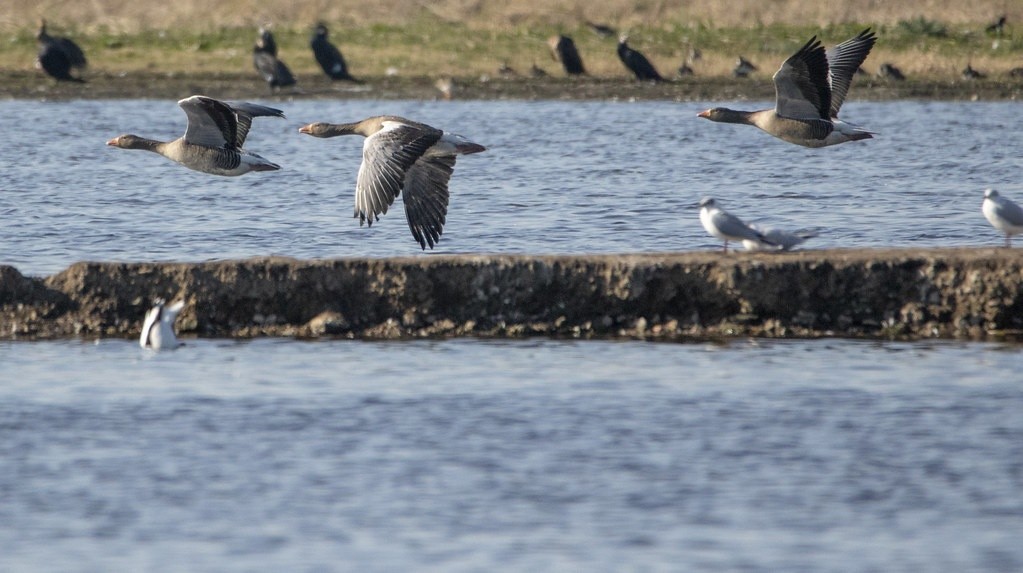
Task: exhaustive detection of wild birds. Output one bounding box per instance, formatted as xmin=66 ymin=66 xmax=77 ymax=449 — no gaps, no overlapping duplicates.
xmin=981 ymin=188 xmax=1023 ymax=248
xmin=311 ymin=23 xmax=367 ymax=88
xmin=697 ymin=28 xmax=879 ymax=148
xmin=34 ymin=14 xmax=90 ymax=84
xmin=299 ymin=115 xmax=486 ymax=250
xmin=139 ymin=296 xmax=185 ymax=349
xmin=107 ymin=95 xmax=287 ymax=176
xmin=698 ymin=195 xmax=821 ymax=252
xmin=252 ymin=23 xmax=298 ymax=96
xmin=617 ymin=33 xmax=662 ymax=80
